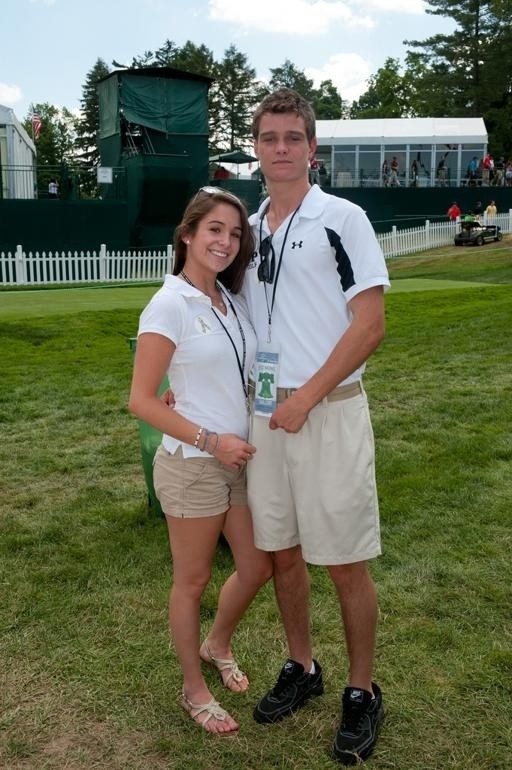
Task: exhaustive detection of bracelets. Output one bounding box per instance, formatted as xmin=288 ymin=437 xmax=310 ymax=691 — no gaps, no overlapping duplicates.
xmin=195 ymin=426 xmax=220 ymax=455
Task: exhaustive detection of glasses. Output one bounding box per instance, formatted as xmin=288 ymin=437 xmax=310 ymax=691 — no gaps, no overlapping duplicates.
xmin=257 ymin=234 xmax=276 ymax=284
xmin=191 ymin=185 xmax=224 ymax=203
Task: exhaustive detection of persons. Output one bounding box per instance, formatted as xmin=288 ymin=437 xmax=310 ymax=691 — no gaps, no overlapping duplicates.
xmin=381 ymin=154 xmax=402 ymax=188
xmin=460 ymin=154 xmax=511 ymax=189
xmin=240 ymin=86 xmax=385 ymax=765
xmin=307 ymin=155 xmax=328 ymax=187
xmin=411 ymin=160 xmax=418 ymax=187
xmin=446 ymin=199 xmax=498 ymax=231
xmin=438 ymin=155 xmax=449 ymax=187
xmin=125 ymin=186 xmax=276 ymax=737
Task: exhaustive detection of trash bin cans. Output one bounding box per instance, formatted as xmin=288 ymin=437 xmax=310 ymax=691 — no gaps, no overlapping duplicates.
xmin=125 ymin=338 xmax=171 ymax=517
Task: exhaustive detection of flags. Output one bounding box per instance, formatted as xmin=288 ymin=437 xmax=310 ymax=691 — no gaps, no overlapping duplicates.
xmin=32 ymin=105 xmax=43 ymax=141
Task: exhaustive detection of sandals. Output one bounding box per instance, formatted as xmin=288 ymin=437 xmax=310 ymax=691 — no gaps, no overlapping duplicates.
xmin=197 ymin=634 xmax=251 ymax=693
xmin=180 ymin=682 xmax=239 ymax=735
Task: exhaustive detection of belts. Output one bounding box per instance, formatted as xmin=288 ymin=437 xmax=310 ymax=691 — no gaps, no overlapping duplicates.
xmin=247 ymin=375 xmax=361 ymax=405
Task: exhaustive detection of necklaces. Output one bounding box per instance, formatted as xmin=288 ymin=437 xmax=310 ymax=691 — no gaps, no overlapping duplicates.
xmin=177 ymin=267 xmax=229 ymax=314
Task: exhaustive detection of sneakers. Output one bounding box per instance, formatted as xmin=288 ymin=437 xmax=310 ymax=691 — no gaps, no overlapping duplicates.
xmin=333 ymin=682 xmax=386 ymax=763
xmin=251 ymin=657 xmax=324 ymax=725
